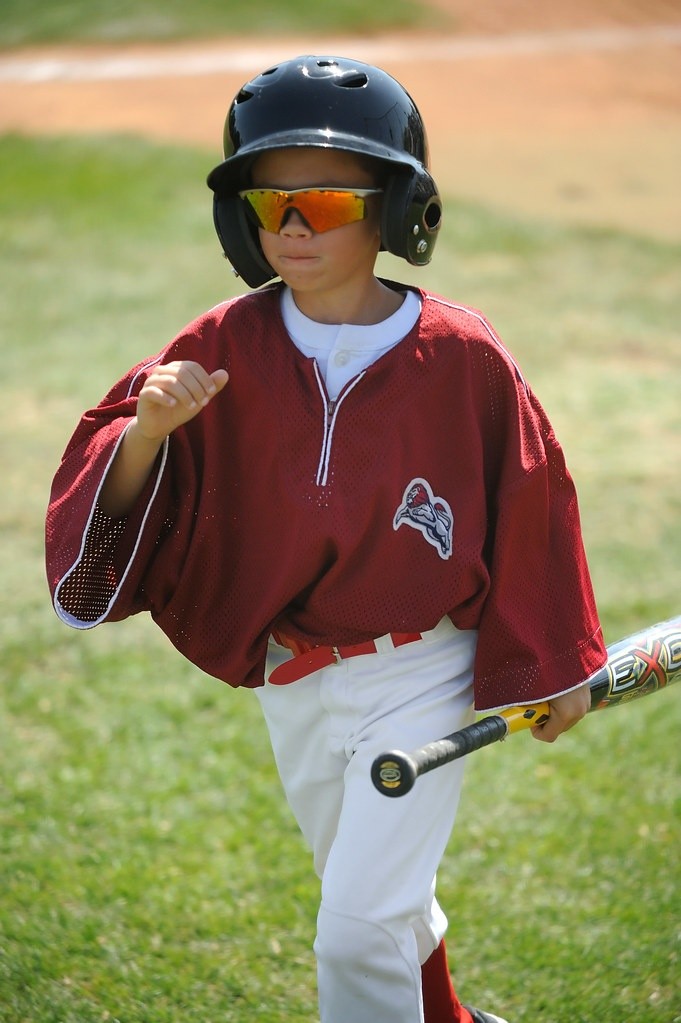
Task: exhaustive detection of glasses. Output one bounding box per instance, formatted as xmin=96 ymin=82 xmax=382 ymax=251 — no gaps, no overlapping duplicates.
xmin=237 ymin=185 xmax=387 ymax=235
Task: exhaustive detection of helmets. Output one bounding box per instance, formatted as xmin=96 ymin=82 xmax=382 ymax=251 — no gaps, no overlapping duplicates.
xmin=205 ymin=55 xmax=442 ymax=289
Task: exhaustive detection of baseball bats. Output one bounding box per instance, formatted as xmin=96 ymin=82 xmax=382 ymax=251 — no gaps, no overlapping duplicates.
xmin=370 ymin=614 xmax=681 ymax=798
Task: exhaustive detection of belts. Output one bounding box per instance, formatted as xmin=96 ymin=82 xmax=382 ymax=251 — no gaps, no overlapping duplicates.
xmin=268 ymin=626 xmax=423 ymax=686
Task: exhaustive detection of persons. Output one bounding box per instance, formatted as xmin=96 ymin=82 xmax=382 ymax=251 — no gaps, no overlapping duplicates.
xmin=46 ymin=56 xmax=607 ymax=1023
